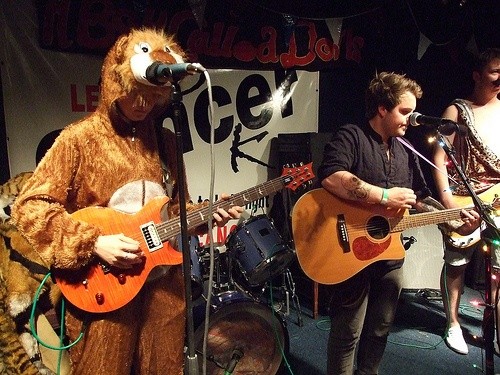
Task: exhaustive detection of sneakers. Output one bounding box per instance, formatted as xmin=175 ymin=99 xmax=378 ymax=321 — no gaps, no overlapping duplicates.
xmin=445 ymin=324 xmax=468 ymax=355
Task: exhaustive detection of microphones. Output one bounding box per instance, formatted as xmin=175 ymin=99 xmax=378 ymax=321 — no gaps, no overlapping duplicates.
xmin=409 ymin=112 xmax=458 ymax=128
xmin=146 ymin=61 xmax=205 ymax=86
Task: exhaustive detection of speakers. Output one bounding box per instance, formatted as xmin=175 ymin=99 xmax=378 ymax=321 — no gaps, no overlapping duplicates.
xmin=278 ymin=132 xmax=334 ymax=237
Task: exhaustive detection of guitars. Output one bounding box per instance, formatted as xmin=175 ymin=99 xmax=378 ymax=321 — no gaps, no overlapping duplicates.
xmin=55 ymin=161 xmax=315 ymax=313
xmin=292 ymin=187 xmax=500 ymax=294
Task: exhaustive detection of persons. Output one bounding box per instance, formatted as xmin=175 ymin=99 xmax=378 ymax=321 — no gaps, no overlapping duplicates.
xmin=315 ymin=69 xmax=482 ymax=375
xmin=9 ymin=26 xmax=244 ymax=374
xmin=432 ymin=48 xmax=500 ymax=356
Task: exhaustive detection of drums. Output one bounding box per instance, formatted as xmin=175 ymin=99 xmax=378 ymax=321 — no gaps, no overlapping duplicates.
xmin=225 ymin=214 xmax=292 ymax=287
xmin=183 ymin=289 xmax=290 ymax=375
xmin=178 ymin=234 xmax=204 ymax=301
